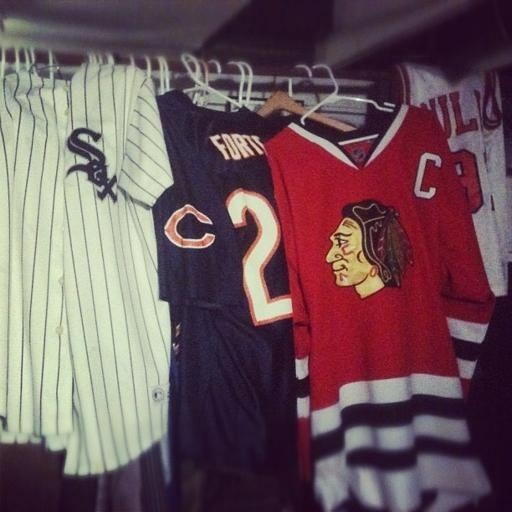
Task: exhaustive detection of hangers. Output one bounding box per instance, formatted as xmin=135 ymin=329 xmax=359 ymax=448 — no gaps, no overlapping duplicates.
xmin=300 ymin=63 xmax=394 ymax=147
xmin=1 ymin=40 xmax=357 ymax=149
